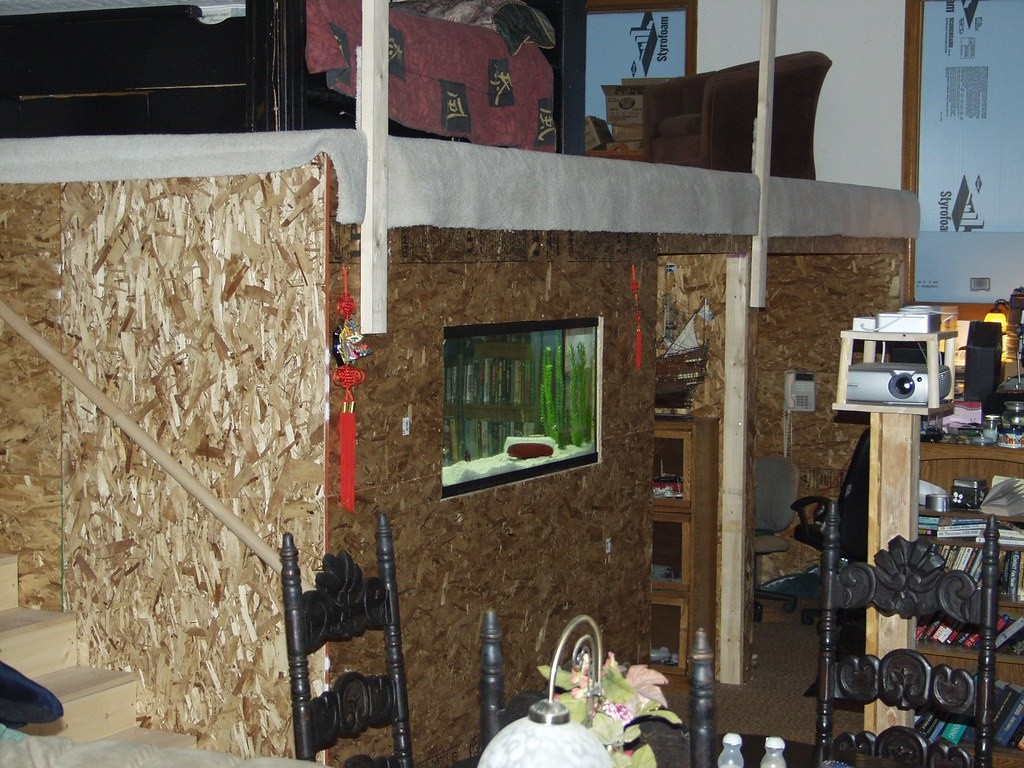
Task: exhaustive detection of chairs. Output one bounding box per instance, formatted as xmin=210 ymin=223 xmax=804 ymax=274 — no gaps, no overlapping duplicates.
xmin=280 ymin=512 xmax=414 ymax=768
xmin=478 ymin=608 xmax=717 ymax=768
xmin=815 ymin=501 xmax=1000 ymax=768
xmin=752 ymin=456 xmax=799 ymax=622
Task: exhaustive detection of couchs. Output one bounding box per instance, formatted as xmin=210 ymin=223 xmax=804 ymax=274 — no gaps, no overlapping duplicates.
xmin=642 ymin=51 xmax=833 ymax=180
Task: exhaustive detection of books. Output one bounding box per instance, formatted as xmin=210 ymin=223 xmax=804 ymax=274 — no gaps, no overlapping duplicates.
xmin=918 ymin=516 xmax=1024 ymax=545
xmin=913 ymin=671 xmax=1024 ymax=750
xmin=980 ymin=475 xmax=1024 ymax=516
xmin=929 ymin=543 xmax=1024 ymax=602
xmin=916 ymin=611 xmax=1024 ymax=656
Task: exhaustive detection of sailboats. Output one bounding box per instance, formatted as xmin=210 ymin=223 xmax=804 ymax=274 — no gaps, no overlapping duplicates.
xmin=653 ymin=458 xmax=683 ymax=499
xmin=655 ymin=262 xmax=714 ymax=423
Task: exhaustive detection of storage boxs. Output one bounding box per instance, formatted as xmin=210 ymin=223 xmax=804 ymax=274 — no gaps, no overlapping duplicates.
xmin=612 ymin=125 xmax=643 ymax=141
xmin=600 ymin=78 xmax=673 ymax=125
xmin=585 ymin=116 xmax=612 ymax=152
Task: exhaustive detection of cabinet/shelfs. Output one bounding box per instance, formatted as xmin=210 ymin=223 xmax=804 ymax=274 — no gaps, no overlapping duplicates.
xmin=915 ymin=442 xmax=1024 ymax=768
xmin=648 ymin=419 xmax=719 ymax=666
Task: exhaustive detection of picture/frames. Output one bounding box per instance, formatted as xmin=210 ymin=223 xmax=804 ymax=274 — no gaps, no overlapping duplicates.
xmin=585 ymin=0 xmax=697 ymax=161
xmin=899 ymin=0 xmax=1024 ymax=322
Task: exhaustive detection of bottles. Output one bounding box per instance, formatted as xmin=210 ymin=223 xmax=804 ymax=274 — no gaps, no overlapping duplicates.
xmin=760 ymin=737 xmax=787 ymax=768
xmin=1002 ymin=401 xmax=1024 ymax=427
xmin=716 ymin=733 xmax=744 ymax=768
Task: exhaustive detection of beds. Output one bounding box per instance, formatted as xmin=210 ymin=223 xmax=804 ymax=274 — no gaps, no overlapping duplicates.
xmin=0 ymin=0 xmax=557 ymax=152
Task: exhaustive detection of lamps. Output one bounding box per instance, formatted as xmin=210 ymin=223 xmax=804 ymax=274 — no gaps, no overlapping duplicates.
xmin=478 ymin=614 xmax=612 ymax=768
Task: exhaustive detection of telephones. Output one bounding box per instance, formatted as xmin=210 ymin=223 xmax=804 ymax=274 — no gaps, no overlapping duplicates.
xmin=783 ymin=370 xmax=816 ymax=412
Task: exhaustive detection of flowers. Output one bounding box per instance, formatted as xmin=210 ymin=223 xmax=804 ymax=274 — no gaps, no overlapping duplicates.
xmin=538 ymin=651 xmax=683 ymax=768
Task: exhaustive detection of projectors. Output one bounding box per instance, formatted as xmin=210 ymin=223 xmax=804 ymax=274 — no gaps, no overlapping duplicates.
xmin=847 ymin=362 xmax=952 ymax=406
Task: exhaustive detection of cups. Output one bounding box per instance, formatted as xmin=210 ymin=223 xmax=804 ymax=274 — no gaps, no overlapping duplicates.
xmin=982 ymin=416 xmax=1001 ymax=443
